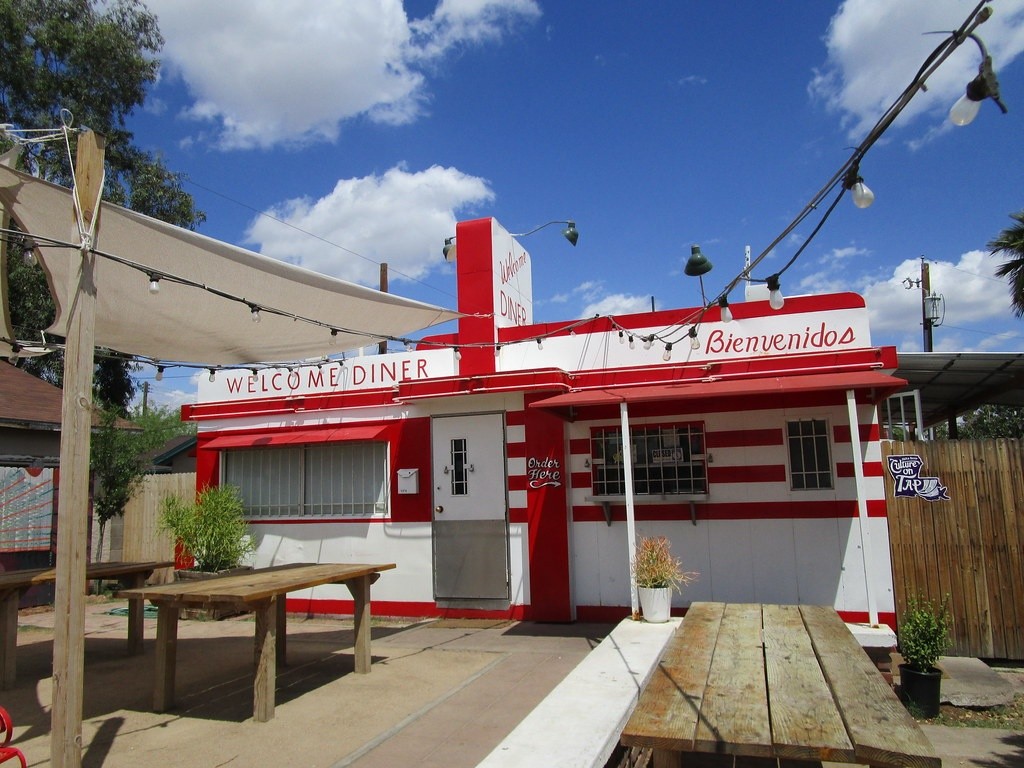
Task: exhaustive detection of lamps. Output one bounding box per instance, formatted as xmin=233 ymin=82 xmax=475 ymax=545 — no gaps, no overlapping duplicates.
xmin=510 ymin=219 xmax=579 ymax=247
xmin=684 ymin=244 xmax=714 ymax=307
xmin=443 ymin=235 xmax=455 ymax=263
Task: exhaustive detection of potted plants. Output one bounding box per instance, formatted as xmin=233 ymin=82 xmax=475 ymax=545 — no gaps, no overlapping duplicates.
xmin=630 ymin=533 xmax=701 ymax=622
xmin=151 ymin=482 xmax=263 ymax=622
xmin=894 ymin=586 xmax=953 ymax=719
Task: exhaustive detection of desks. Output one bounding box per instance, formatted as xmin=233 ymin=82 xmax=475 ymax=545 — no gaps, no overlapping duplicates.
xmin=0 ymin=561 xmax=176 ymax=692
xmin=620 ymin=602 xmax=943 ymax=768
xmin=111 ymin=562 xmax=399 ymax=722
